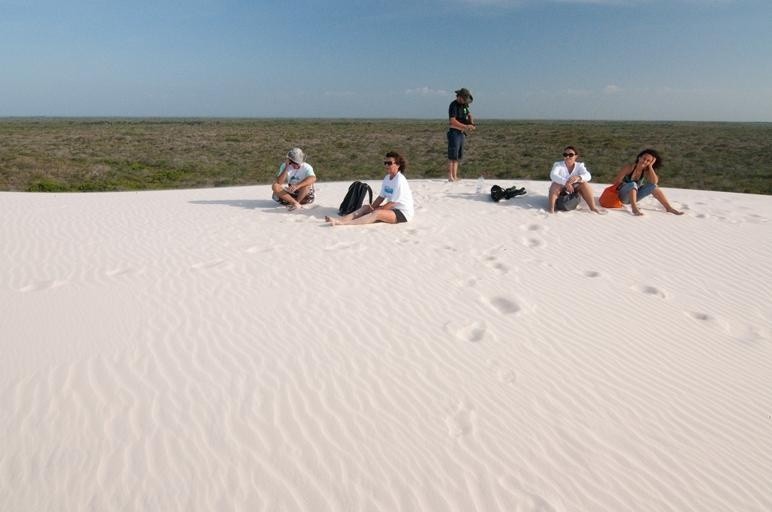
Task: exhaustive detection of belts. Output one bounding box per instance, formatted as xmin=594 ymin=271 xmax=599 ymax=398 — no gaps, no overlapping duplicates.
xmin=450 ymin=128 xmax=468 ymax=136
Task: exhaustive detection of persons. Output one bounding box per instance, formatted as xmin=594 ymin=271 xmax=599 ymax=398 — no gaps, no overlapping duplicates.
xmin=548 ymin=144 xmax=601 ymax=216
xmin=445 ymin=88 xmax=476 ymax=182
xmin=324 ymin=150 xmax=416 ymax=226
xmin=614 ymin=148 xmax=685 ymax=216
xmin=268 ymin=147 xmax=316 ymax=210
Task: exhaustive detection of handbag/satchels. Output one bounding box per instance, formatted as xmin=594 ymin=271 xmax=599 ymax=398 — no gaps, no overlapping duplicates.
xmin=598 ymin=185 xmax=624 ymax=208
xmin=556 ymin=191 xmax=582 ymax=211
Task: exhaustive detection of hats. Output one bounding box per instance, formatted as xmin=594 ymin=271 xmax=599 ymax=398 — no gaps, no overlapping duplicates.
xmin=454 ymin=87 xmax=473 ymax=104
xmin=286 ymin=147 xmax=307 ymax=165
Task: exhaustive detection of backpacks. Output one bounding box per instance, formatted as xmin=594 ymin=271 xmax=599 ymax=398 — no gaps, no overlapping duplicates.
xmin=338 ymin=179 xmax=373 ymax=217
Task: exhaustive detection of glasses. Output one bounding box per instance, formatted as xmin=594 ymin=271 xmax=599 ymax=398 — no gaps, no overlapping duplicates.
xmin=383 ymin=160 xmax=395 ymax=166
xmin=563 ymin=153 xmax=574 ymax=157
